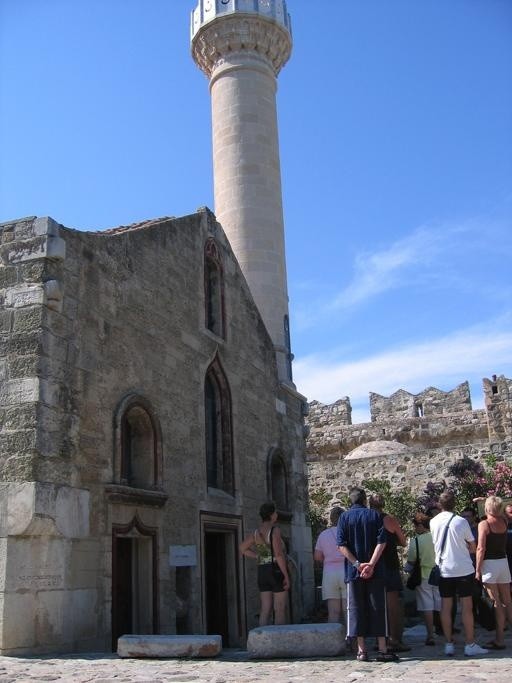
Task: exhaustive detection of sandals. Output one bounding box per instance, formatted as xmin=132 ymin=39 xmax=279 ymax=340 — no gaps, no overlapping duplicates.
xmin=425 ymin=636 xmax=436 ymax=645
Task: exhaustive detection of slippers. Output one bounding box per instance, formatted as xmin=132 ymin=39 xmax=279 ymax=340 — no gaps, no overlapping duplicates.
xmin=481 ymin=640 xmax=506 ymax=650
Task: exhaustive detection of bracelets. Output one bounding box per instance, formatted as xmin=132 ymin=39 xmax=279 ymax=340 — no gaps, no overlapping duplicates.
xmin=353 ymin=559 xmax=360 ymax=567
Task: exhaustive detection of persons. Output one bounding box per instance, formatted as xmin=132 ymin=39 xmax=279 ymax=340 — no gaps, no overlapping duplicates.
xmin=431 ymin=502 xmax=512 ymax=635
xmin=403 ymin=511 xmax=443 ymax=646
xmin=336 ymin=486 xmax=399 ymax=662
xmin=367 ymin=492 xmax=411 ymax=651
xmin=313 ymin=505 xmax=349 ymax=622
xmin=473 ymin=496 xmax=512 ymax=650
xmin=237 ymin=502 xmax=291 ymax=625
xmin=430 ymin=491 xmax=490 ymax=656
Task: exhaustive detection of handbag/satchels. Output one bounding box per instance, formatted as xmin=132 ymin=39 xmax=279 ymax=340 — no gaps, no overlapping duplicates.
xmin=474 ymin=596 xmax=497 ymax=631
xmin=404 ymin=558 xmax=422 ymax=591
xmin=427 ymin=564 xmax=441 ymax=587
xmin=285 ymin=554 xmax=298 ymax=578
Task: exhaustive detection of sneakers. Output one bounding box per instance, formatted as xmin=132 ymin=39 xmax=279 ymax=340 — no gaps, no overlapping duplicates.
xmin=375 ymin=649 xmax=399 ymax=663
xmin=444 ymin=640 xmax=457 ymax=657
xmin=463 ymin=641 xmax=489 ymax=657
xmin=356 ymin=651 xmax=369 ymax=661
xmin=386 ymin=638 xmax=412 ymax=653
